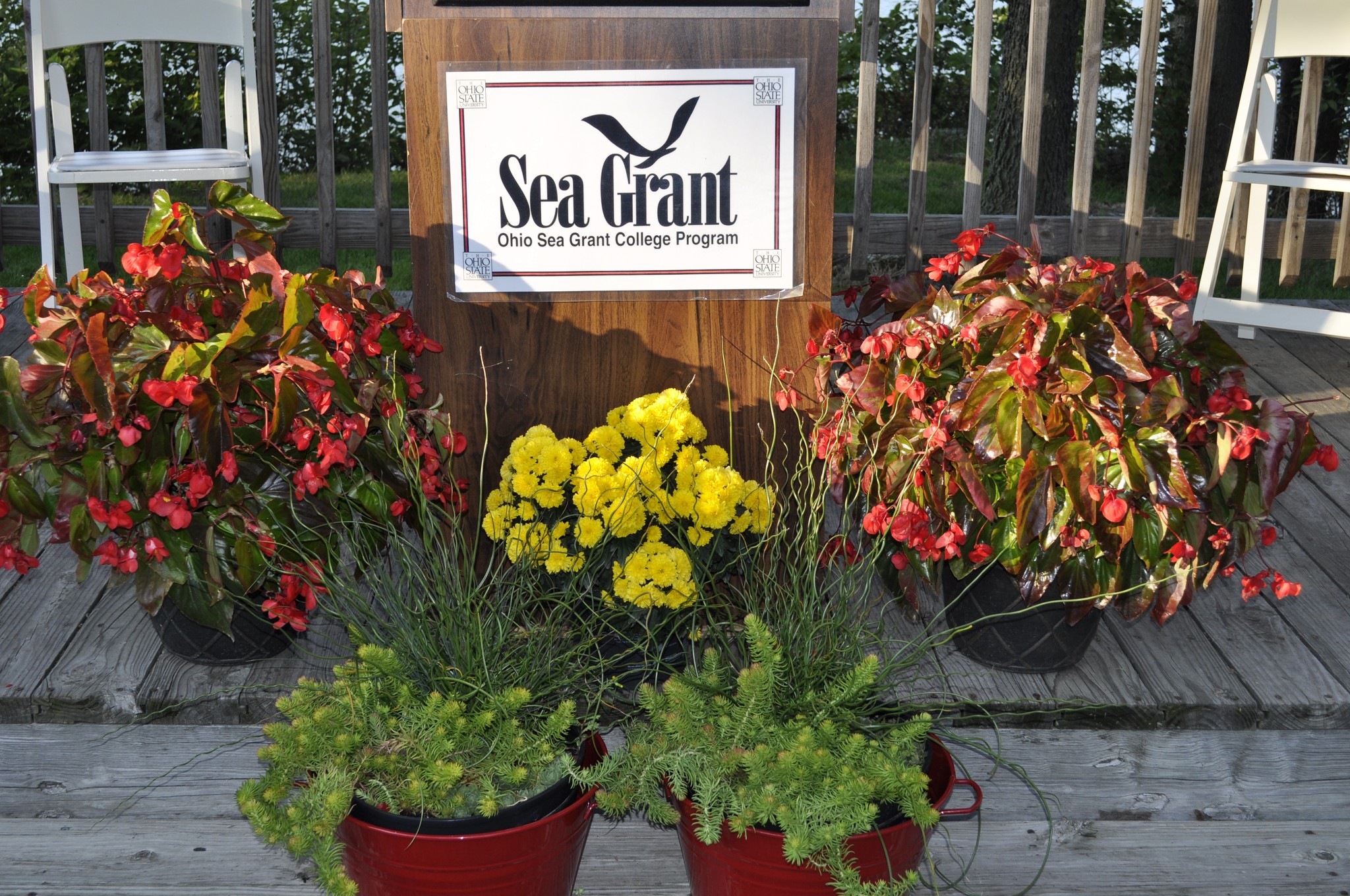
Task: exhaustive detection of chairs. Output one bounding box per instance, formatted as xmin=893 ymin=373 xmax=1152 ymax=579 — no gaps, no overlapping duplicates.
xmin=21 ymin=1 xmax=269 ymax=312
xmin=1185 ymin=0 xmax=1350 ymax=352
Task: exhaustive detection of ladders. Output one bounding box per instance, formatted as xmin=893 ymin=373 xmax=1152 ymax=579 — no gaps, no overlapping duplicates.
xmin=30 ymin=0 xmax=268 ymax=367
xmin=1192 ymin=1 xmax=1350 ymax=352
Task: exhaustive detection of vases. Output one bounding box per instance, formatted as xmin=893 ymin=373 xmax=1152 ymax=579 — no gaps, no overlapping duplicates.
xmin=131 ymin=525 xmax=332 ymax=664
xmin=944 ymin=556 xmax=1103 ymax=671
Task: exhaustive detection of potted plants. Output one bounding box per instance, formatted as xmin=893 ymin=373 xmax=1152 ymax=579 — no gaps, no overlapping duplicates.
xmin=594 ymin=350 xmax=1190 ymax=896
xmin=71 ymin=336 xmax=672 ymax=895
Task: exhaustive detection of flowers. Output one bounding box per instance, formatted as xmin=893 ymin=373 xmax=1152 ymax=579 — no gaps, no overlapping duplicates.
xmin=480 ymin=386 xmax=775 ymax=637
xmin=778 ymin=221 xmax=1342 ymax=629
xmin=2 ymin=184 xmax=468 ymax=633
